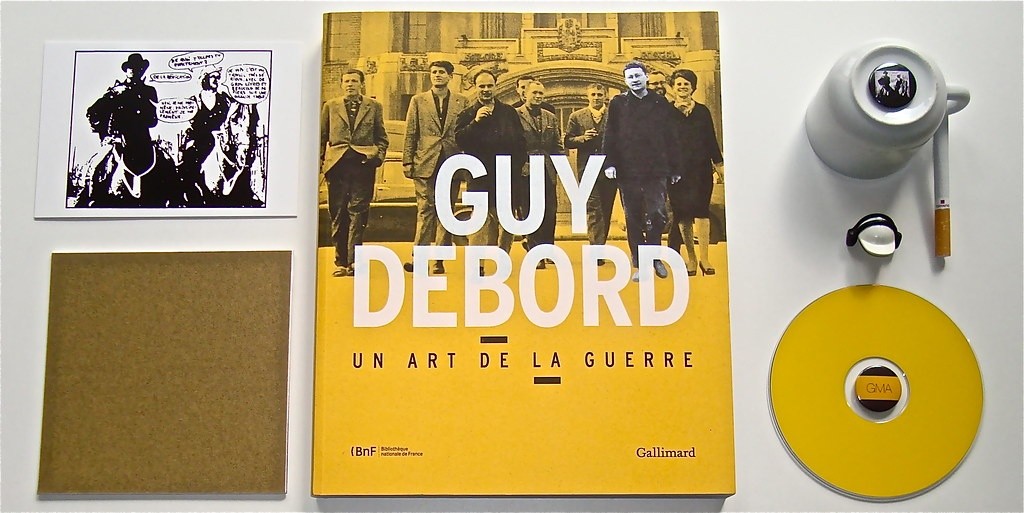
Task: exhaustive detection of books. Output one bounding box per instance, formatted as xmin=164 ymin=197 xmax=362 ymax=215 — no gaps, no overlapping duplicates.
xmin=308 ymin=11 xmax=736 ymax=503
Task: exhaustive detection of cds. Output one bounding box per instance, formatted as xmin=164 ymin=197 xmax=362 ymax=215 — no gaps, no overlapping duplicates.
xmin=766 ymin=284 xmax=985 ymax=503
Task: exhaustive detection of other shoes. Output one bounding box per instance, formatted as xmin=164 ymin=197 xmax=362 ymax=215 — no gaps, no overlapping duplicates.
xmin=432 ymin=261 xmax=446 ymax=273
xmin=480 ymin=267 xmax=485 ymax=276
xmin=630 ymin=269 xmax=639 ymax=282
xmin=403 ymin=262 xmax=413 ymax=272
xmin=537 ymin=258 xmax=554 ymax=269
xmin=331 ymin=265 xmax=355 ymax=277
xmin=654 ymin=260 xmax=668 ymax=278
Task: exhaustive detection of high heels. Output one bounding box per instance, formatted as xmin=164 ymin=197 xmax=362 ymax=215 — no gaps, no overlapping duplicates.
xmin=686 ymin=258 xmax=715 ymax=276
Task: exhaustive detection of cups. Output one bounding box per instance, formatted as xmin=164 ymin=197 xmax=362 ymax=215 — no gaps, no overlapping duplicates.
xmin=805 ymin=37 xmax=970 ymax=180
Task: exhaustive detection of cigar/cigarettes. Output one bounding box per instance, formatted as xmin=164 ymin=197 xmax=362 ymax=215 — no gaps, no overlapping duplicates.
xmin=933 ymin=114 xmax=952 ymax=259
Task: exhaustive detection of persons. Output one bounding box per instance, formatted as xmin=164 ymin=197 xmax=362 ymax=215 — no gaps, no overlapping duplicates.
xmin=318 ymin=68 xmax=389 ymax=277
xmin=665 ymin=69 xmax=725 ymax=279
xmin=878 ymin=71 xmax=891 ymax=95
xmin=891 ymin=74 xmax=906 ymax=94
xmin=512 ymin=80 xmax=568 ymax=271
xmin=510 ymin=76 xmax=557 ymax=113
xmin=403 ymin=59 xmax=470 ymax=278
xmin=452 ymin=69 xmax=528 ymax=282
xmin=563 ymin=83 xmax=617 ymax=266
xmin=601 ymin=59 xmax=683 ymax=282
xmin=658 ymin=75 xmax=684 ymax=258
